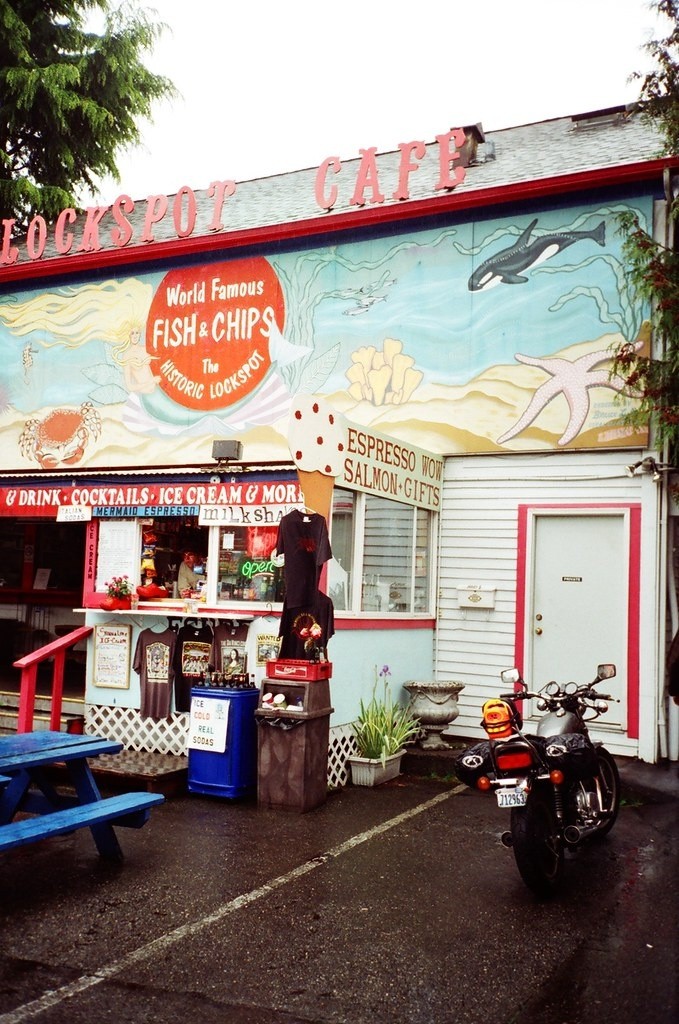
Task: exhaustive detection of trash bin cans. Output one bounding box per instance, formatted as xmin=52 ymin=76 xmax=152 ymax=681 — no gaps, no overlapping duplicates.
xmin=253 ymin=678 xmax=335 ymax=812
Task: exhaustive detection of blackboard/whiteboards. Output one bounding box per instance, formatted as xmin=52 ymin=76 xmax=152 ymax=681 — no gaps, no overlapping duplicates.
xmin=93 ymin=623 xmax=132 ymax=689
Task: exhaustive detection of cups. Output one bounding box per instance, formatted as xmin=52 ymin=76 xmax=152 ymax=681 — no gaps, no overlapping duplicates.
xmin=274 ymin=694 xmax=287 ymax=709
xmin=262 ymin=693 xmax=276 ymax=707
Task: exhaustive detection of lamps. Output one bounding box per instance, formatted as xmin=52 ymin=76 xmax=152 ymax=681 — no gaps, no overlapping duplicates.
xmin=651 ymin=458 xmax=664 ymax=482
xmin=625 ymin=460 xmax=643 ymax=477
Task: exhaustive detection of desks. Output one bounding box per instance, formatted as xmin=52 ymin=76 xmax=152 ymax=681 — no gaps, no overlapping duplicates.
xmin=0 ymin=729 xmax=125 ymax=862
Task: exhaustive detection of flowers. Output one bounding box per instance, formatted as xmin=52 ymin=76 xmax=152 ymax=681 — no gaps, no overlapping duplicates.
xmin=351 ymin=665 xmax=420 ymax=771
xmin=105 ymin=574 xmax=135 ymax=599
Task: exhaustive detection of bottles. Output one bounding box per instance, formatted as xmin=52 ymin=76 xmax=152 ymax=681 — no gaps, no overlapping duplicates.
xmin=314 ymin=647 xmax=319 ymax=663
xmin=233 ymin=578 xmax=260 ymax=600
xmin=260 ymin=577 xmax=268 ymax=601
xmin=268 ymin=577 xmax=276 ymax=603
xmin=219 ymin=674 xmax=226 ymax=687
xmin=226 ymin=673 xmax=250 ymax=689
xmin=212 ymin=673 xmax=218 ymax=687
xmin=250 ymin=674 xmax=256 ymax=688
xmin=204 ymin=672 xmax=211 ymax=686
xmin=319 ymin=647 xmax=325 ymax=663
xmin=275 ymin=567 xmax=284 ymax=602
xmin=197 ymin=671 xmax=204 ymax=686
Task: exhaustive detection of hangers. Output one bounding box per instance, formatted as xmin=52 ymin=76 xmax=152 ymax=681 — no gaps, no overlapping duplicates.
xmin=297 ymin=492 xmax=316 ymax=515
xmin=190 ymin=618 xmax=207 ymax=629
xmin=262 ymin=602 xmax=279 ymax=622
xmin=150 ymin=616 xmax=167 ymax=629
xmin=226 ymin=619 xmax=244 ymax=628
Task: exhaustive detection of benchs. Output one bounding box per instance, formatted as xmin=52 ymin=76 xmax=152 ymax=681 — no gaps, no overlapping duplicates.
xmin=0 ymin=791 xmax=165 ymax=853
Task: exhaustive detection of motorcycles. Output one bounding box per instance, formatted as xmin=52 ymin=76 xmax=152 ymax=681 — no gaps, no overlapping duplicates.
xmin=479 ymin=663 xmax=625 ymax=896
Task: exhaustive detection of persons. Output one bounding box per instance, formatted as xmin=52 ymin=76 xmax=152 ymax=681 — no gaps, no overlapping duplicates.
xmin=178 ymin=534 xmax=208 ymax=599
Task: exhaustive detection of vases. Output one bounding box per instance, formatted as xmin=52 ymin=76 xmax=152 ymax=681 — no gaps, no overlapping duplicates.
xmin=402 ymin=680 xmax=466 ymax=749
xmin=119 ymin=593 xmax=132 ymax=608
xmin=347 ymin=748 xmax=407 ymax=786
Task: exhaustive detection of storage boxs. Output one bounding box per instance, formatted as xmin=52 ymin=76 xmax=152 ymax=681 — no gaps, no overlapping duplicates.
xmin=266 ymin=658 xmax=332 ymax=681
